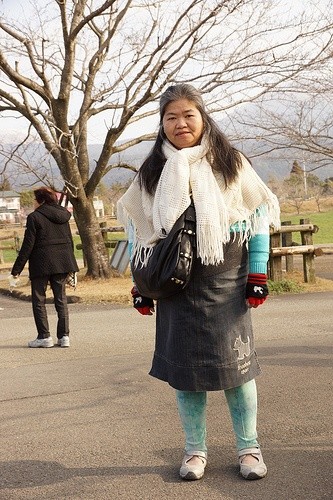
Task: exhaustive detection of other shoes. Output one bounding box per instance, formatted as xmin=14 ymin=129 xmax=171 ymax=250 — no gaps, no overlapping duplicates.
xmin=180 ymin=450 xmax=207 ymax=480
xmin=238 ymin=448 xmax=267 ymax=479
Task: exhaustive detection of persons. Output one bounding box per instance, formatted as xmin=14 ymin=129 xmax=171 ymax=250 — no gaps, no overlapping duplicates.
xmin=11 ymin=187 xmax=79 ymax=348
xmin=116 ymin=83 xmax=281 ymax=480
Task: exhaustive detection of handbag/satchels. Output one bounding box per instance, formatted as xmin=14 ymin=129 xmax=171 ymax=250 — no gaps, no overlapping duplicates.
xmin=65 ymin=272 xmax=78 ymax=290
xmin=132 ymin=215 xmax=193 ymax=298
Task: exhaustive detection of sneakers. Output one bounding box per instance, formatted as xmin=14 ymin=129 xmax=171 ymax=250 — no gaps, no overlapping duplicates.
xmin=58 ymin=336 xmax=70 ymax=347
xmin=29 ymin=337 xmax=54 ymax=347
